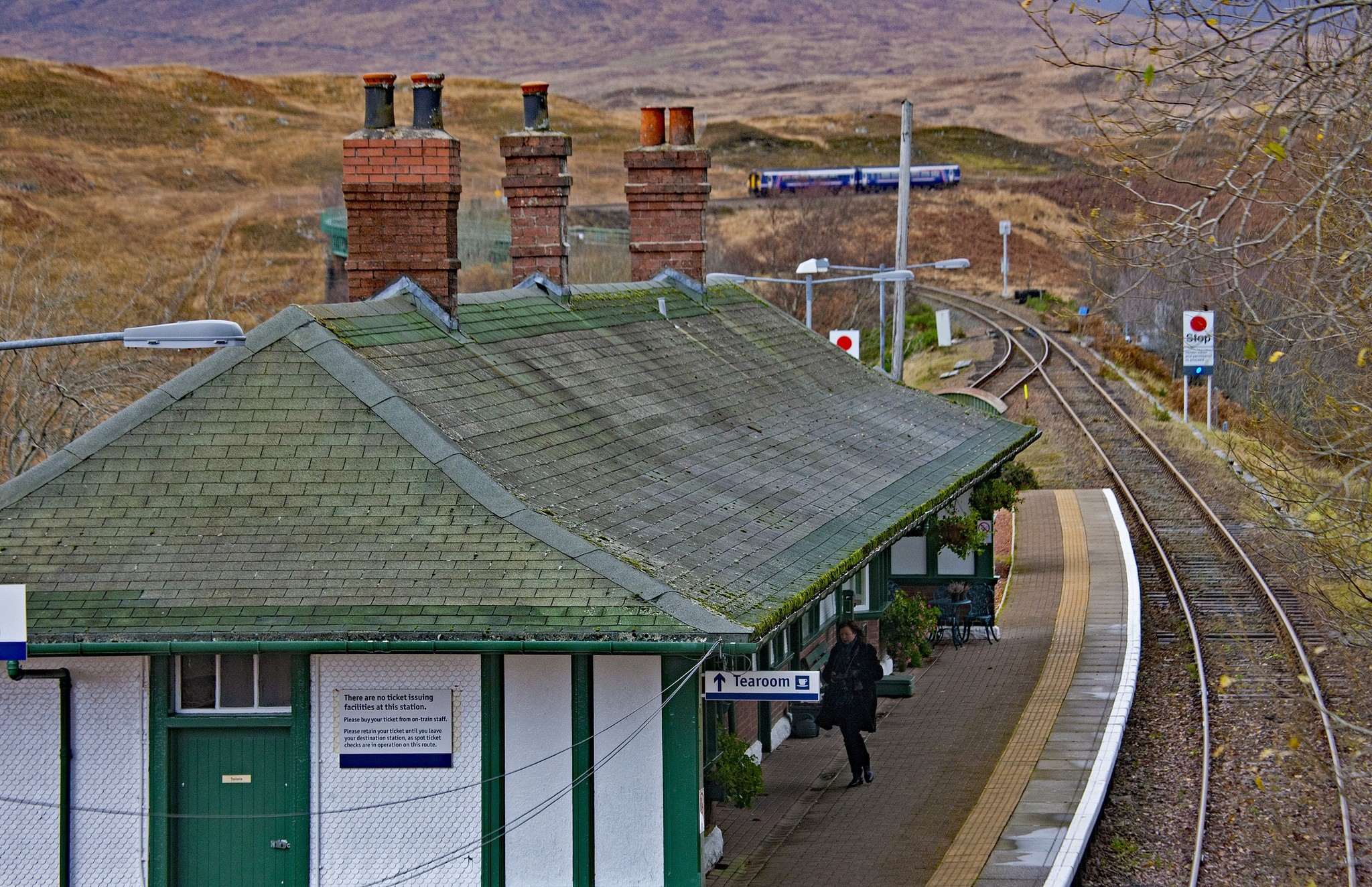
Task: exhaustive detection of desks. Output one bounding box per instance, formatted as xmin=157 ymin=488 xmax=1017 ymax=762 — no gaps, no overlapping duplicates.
xmin=927 ymin=598 xmax=972 ymax=651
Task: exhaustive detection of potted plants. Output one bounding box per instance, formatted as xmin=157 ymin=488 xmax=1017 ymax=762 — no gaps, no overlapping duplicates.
xmin=926 ymin=459 xmax=1045 ymax=562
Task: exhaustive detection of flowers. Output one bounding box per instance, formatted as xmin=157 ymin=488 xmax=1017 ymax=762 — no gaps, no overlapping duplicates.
xmin=947 ymin=580 xmax=972 ymax=593
xmin=877 ymin=588 xmax=942 ymax=672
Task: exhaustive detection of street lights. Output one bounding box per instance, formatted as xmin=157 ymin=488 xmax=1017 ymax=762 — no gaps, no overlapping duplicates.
xmin=706 ymin=270 xmax=914 ymax=330
xmin=796 ymin=258 xmax=971 ymax=370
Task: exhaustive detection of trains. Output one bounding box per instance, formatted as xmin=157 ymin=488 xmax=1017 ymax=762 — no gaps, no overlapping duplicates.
xmin=746 ymin=164 xmax=961 ymax=198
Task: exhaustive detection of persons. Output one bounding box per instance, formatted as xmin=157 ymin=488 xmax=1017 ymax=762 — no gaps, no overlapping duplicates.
xmin=821 ymin=620 xmax=884 ymax=788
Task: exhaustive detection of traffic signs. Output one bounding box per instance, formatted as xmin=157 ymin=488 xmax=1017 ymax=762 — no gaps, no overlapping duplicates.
xmin=705 ymin=671 xmax=820 ymax=702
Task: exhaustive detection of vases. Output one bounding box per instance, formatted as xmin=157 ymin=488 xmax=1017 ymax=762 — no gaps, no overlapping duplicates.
xmin=951 ymin=593 xmax=960 ymax=602
xmin=960 ymin=593 xmax=964 ymax=600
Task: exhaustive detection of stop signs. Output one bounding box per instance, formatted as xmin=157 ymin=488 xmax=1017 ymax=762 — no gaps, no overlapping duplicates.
xmin=1183 ymin=310 xmax=1213 ymax=373
xmin=829 ymin=330 xmax=859 ymax=360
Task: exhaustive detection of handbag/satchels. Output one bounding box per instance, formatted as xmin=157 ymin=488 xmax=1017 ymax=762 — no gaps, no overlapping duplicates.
xmin=814 ymin=693 xmax=838 ymax=731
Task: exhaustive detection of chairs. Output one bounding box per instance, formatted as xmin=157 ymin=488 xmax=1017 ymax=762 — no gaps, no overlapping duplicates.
xmin=961 ymin=582 xmax=999 ymax=646
xmin=927 ymin=584 xmax=963 ymax=642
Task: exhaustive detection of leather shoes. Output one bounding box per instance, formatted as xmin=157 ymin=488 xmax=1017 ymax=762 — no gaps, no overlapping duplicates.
xmin=863 ymin=766 xmax=874 ymax=783
xmin=848 ymin=773 xmax=863 ymax=787
